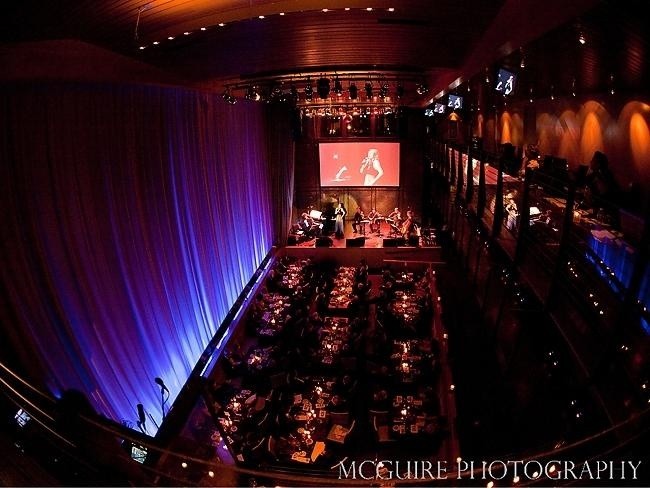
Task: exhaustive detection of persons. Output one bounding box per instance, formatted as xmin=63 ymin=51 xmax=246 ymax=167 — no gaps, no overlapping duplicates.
xmin=360 ymin=148 xmax=384 ymax=186
xmin=185 ymin=251 xmax=451 ymax=479
xmin=465 ymin=129 xmax=649 ymax=263
xmin=290 ymin=196 xmax=453 ymax=248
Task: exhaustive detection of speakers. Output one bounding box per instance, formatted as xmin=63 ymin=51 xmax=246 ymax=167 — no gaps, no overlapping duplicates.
xmin=315 ymin=235 xmax=419 ymax=247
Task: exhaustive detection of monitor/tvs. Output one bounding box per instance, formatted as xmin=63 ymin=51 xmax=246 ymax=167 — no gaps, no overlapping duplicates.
xmin=492 ymin=65 xmax=519 ymax=97
xmin=446 ymin=92 xmax=464 ymax=112
xmin=317 ymin=138 xmax=401 ymax=189
xmin=423 ymin=107 xmax=435 ymax=117
xmin=432 ymin=102 xmax=447 ymax=115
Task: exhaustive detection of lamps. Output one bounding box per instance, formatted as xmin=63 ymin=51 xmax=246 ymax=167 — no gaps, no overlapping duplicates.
xmin=217 ymin=70 xmax=405 ymax=103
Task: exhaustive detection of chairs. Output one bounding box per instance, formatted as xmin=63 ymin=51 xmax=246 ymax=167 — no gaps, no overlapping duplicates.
xmin=190 ymin=258 xmax=442 ymax=472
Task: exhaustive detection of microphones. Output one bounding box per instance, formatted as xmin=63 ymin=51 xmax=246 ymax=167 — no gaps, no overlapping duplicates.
xmin=155 ymin=377 xmax=169 ymax=392
xmin=136 ymin=403 xmax=146 ymax=431
